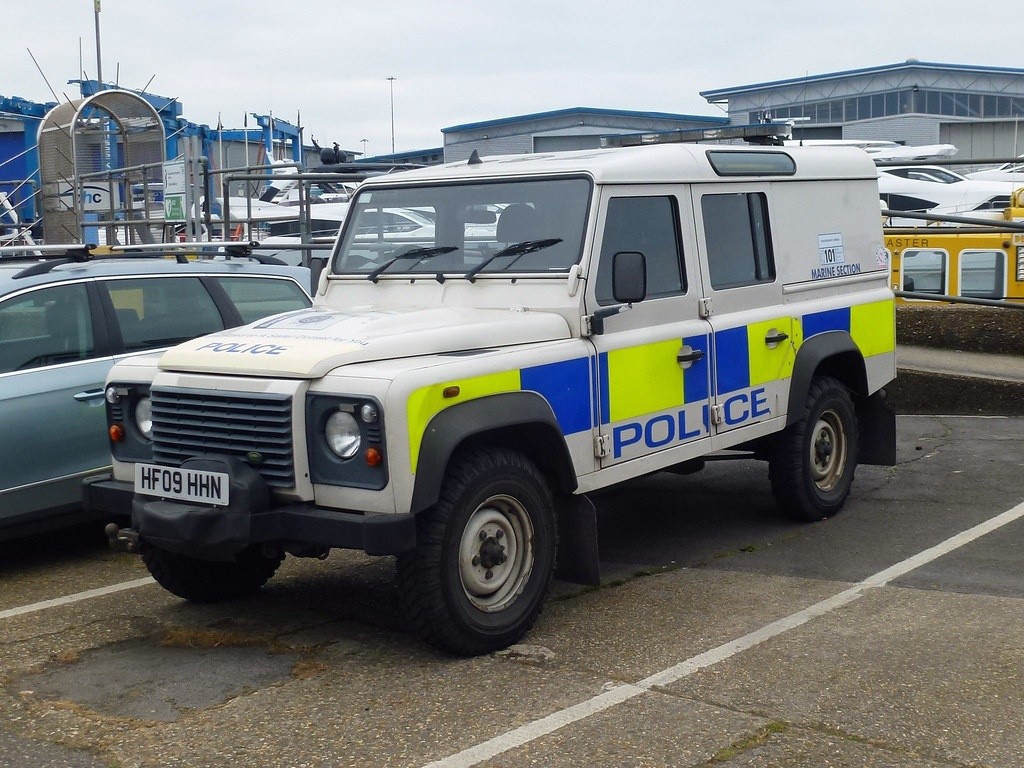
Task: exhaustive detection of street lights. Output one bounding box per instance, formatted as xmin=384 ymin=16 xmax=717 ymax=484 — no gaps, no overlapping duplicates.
xmin=360 ymin=138 xmax=369 ymax=159
xmin=387 ymin=74 xmax=397 ymax=153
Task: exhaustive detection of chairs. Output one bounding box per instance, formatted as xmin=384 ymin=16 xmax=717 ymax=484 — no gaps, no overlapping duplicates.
xmin=479 ymin=201 xmax=551 ymax=270
xmin=44 ymin=303 xmax=79 ymax=352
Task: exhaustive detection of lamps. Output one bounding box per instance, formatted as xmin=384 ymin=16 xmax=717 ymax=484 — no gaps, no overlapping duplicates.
xmin=482 ymin=135 xmax=488 ymax=139
xmin=578 ymin=121 xmax=584 ymax=125
xmin=912 ymin=84 xmax=919 ymax=91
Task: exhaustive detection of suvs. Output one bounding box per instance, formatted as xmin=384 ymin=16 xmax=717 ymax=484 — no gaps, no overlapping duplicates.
xmin=78 ymin=120 xmax=900 ymax=655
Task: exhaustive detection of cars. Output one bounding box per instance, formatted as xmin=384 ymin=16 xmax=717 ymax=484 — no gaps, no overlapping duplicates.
xmin=0 ymin=243 xmax=319 ymax=552
xmin=191 ymin=182 xmax=529 ymax=274
xmin=784 ymin=139 xmax=1024 ymax=295
